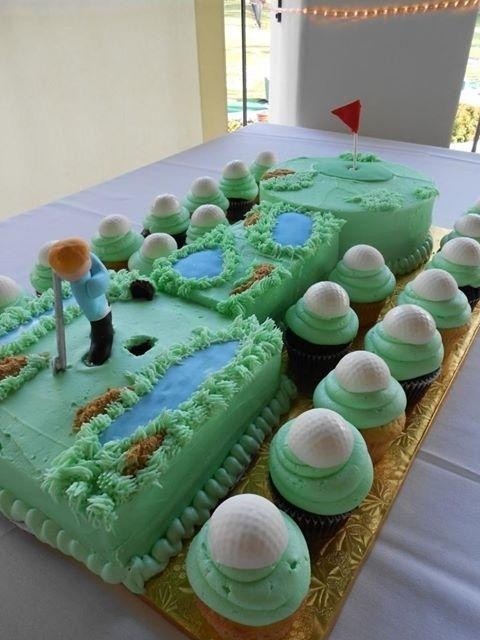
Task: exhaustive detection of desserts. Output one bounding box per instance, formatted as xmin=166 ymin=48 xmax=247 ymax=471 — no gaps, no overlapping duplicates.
xmin=1 ymin=150 xmax=480 ymax=639
